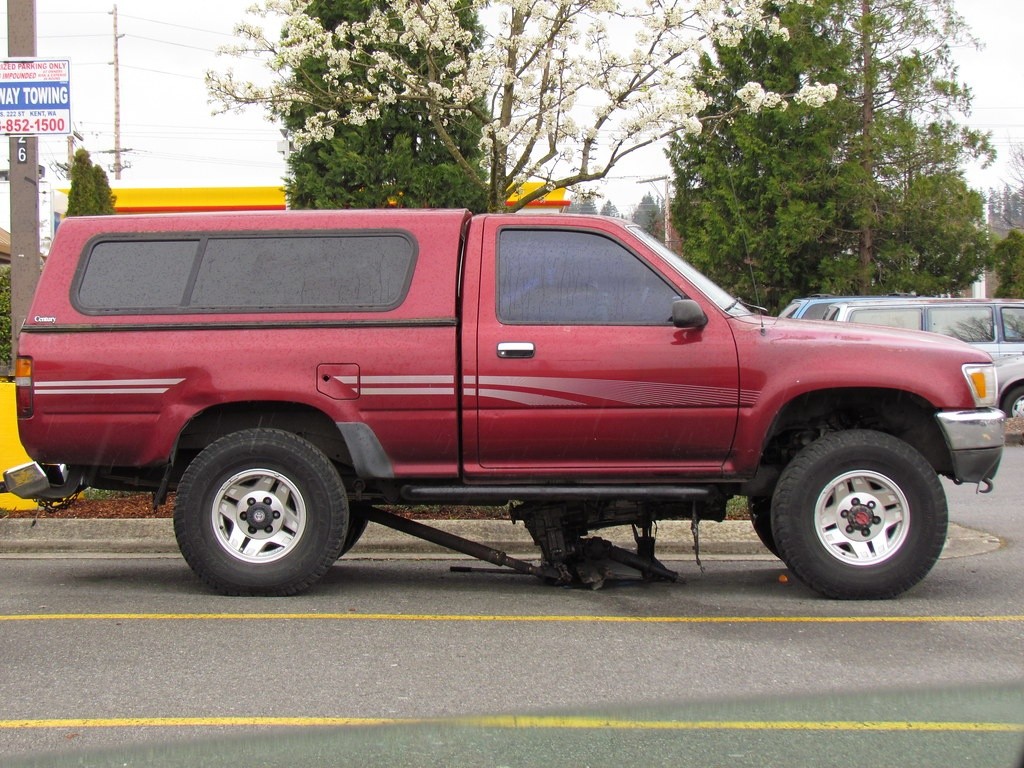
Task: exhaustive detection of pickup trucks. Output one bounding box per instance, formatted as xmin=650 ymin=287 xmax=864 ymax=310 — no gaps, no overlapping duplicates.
xmin=1 ymin=204 xmax=1008 ymax=603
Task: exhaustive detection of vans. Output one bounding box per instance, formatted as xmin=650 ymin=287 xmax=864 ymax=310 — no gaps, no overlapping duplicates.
xmin=779 ymin=291 xmax=1024 ymax=422
